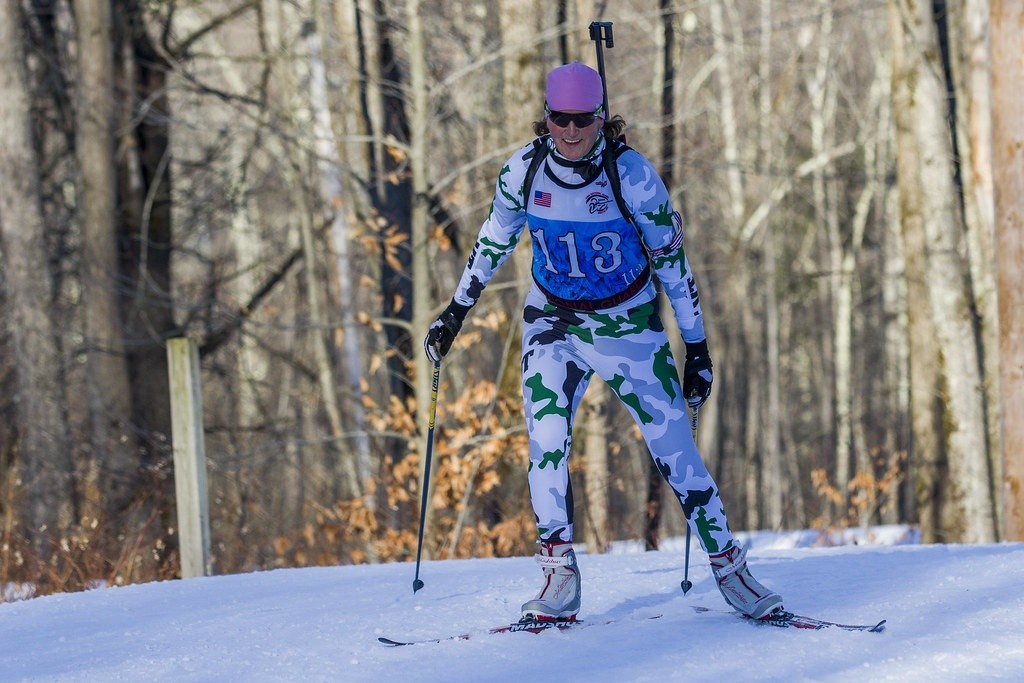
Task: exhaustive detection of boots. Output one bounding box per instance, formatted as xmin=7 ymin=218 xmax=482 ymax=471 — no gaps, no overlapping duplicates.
xmin=521 ymin=541 xmax=581 ymax=621
xmin=709 ymin=543 xmax=784 ymax=619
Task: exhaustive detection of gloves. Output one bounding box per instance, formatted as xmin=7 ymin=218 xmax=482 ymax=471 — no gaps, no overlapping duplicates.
xmin=423 ymin=296 xmax=472 ymax=362
xmin=683 ymin=338 xmax=713 ymax=410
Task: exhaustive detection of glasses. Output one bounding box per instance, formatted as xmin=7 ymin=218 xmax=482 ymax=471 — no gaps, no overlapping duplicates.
xmin=544 ymin=103 xmax=602 ymax=128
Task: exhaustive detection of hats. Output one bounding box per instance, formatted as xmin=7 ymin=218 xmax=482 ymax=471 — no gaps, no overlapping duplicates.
xmin=545 ymin=60 xmax=605 ymax=120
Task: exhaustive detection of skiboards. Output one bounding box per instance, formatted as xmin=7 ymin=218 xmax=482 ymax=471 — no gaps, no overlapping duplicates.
xmin=376 ymin=600 xmax=890 ymax=647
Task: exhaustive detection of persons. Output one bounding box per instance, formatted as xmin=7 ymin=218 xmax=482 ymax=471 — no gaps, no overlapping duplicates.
xmin=425 ymin=61 xmax=785 ymax=620
